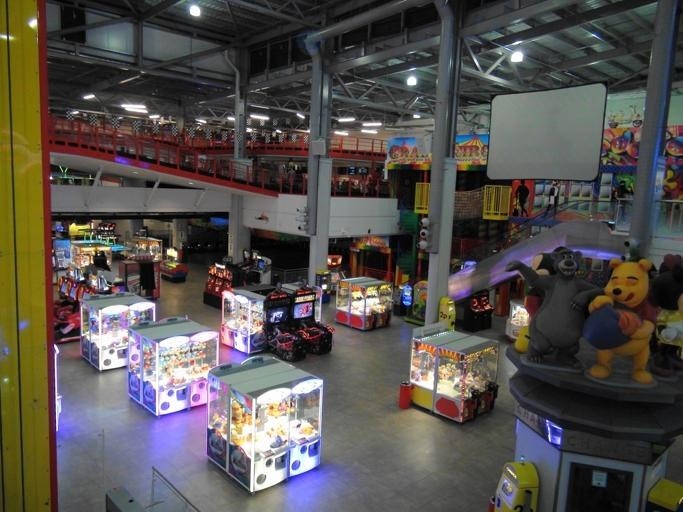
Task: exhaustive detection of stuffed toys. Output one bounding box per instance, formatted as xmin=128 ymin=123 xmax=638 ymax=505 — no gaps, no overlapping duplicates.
xmin=412 ymin=347 xmax=496 ymax=397
xmin=515 ymin=253 xmax=555 ymax=352
xmin=208 ymin=393 xmax=316 ymax=462
xmin=649 ymin=254 xmax=683 ymax=375
xmin=507 ymin=247 xmax=600 ymax=369
xmin=130 ymin=339 xmax=211 ymax=391
xmin=579 ymin=257 xmax=656 ymax=384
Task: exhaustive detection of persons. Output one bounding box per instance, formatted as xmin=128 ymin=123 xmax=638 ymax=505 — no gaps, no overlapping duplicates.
xmin=611 ymin=180 xmax=633 ymax=222
xmin=513 ymin=180 xmax=531 ymax=218
xmin=543 ymin=182 xmax=559 ymax=220
